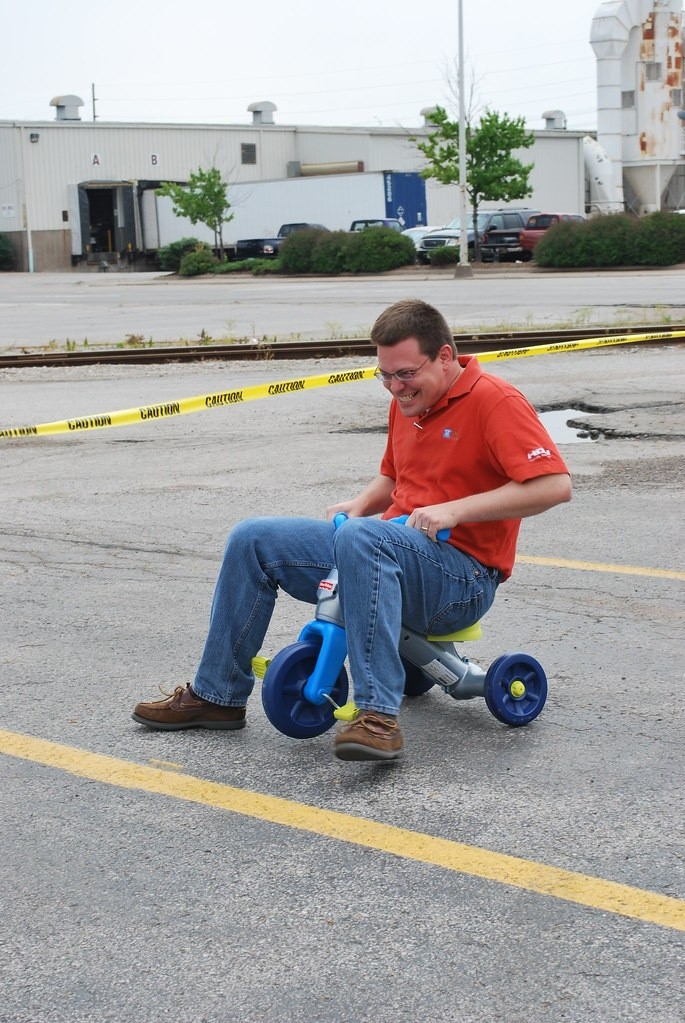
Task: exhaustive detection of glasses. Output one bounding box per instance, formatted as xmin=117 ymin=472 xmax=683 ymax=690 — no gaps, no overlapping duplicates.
xmin=374 ymin=351 xmax=435 ymax=382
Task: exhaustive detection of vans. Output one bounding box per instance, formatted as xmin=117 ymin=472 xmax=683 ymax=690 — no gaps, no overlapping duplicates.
xmin=519 ymin=212 xmax=589 ymax=252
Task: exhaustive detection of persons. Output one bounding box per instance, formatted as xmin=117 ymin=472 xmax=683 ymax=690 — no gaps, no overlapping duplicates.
xmin=131 ymin=299 xmax=571 ymax=764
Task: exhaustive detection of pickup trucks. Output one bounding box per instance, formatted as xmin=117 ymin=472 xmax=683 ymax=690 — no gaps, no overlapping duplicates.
xmin=234 ymin=222 xmax=332 ymax=260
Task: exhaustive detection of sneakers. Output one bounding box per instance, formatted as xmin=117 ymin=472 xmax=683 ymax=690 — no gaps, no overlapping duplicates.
xmin=334 ymin=708 xmax=405 ymax=761
xmin=132 ymin=682 xmax=247 ymax=730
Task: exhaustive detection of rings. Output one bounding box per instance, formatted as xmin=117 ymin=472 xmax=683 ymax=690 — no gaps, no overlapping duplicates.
xmin=420 ymin=526 xmax=428 ymax=531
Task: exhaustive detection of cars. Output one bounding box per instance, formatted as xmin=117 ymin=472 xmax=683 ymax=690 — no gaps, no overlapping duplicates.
xmin=399 ymin=225 xmax=445 ymax=261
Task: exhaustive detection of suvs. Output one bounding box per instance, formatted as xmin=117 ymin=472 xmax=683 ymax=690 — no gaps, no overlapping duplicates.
xmin=346 ymin=218 xmax=406 ymax=237
xmin=415 ymin=207 xmax=543 ymax=266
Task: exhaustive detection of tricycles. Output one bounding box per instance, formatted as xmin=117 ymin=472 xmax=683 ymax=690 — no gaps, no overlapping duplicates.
xmin=252 ymin=511 xmax=549 ymax=741
